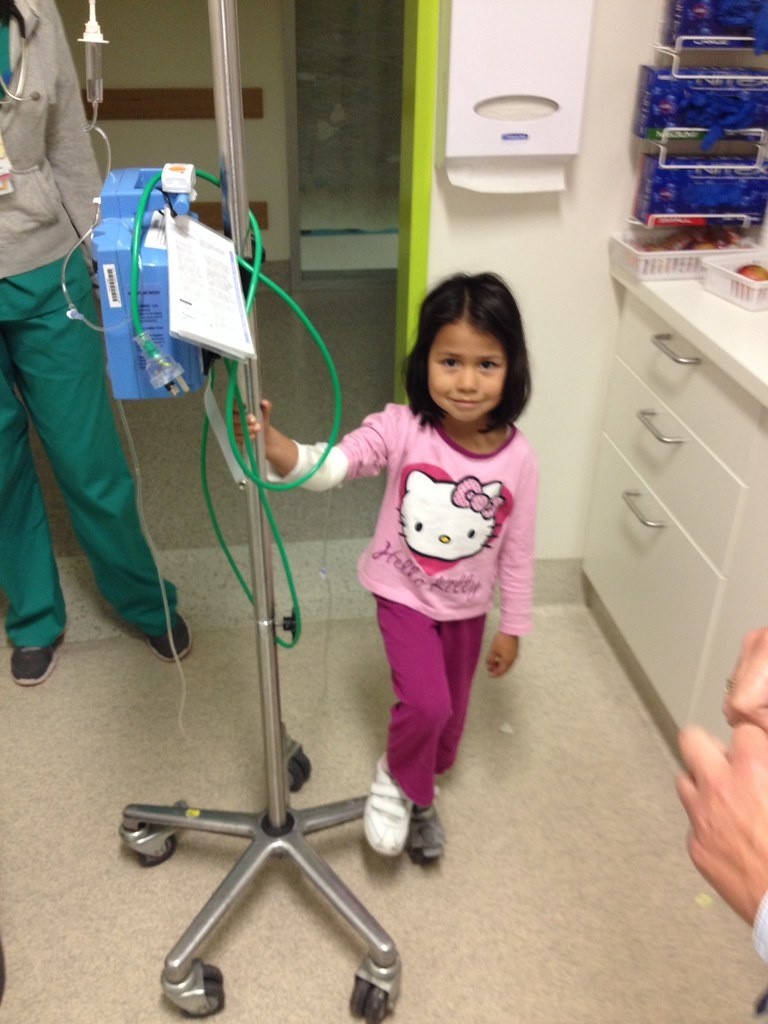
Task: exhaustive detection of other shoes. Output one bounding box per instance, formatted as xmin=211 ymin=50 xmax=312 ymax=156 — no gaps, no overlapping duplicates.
xmin=11 ymin=629 xmax=64 ymax=685
xmin=141 ymin=611 xmax=192 ymax=661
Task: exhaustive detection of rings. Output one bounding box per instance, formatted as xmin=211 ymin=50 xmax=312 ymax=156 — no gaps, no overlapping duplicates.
xmin=724 ymin=678 xmax=735 ymax=694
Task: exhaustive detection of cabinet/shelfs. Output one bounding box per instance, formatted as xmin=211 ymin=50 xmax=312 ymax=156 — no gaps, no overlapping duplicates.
xmin=626 ymin=0 xmax=768 ymax=229
xmin=582 ymin=292 xmax=768 ymax=784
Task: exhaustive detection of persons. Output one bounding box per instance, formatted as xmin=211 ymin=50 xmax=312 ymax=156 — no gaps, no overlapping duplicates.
xmin=233 ymin=273 xmax=538 ymax=855
xmin=676 ymin=625 xmax=768 ymax=961
xmin=0 ymin=0 xmax=190 ymax=687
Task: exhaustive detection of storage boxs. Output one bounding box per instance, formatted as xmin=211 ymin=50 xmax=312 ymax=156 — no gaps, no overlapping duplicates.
xmin=611 ymin=228 xmax=761 ymax=282
xmin=698 ymin=251 xmax=768 ymax=312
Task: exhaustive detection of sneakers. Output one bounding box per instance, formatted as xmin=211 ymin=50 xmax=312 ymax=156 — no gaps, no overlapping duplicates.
xmin=363 ymin=751 xmax=414 ymax=857
